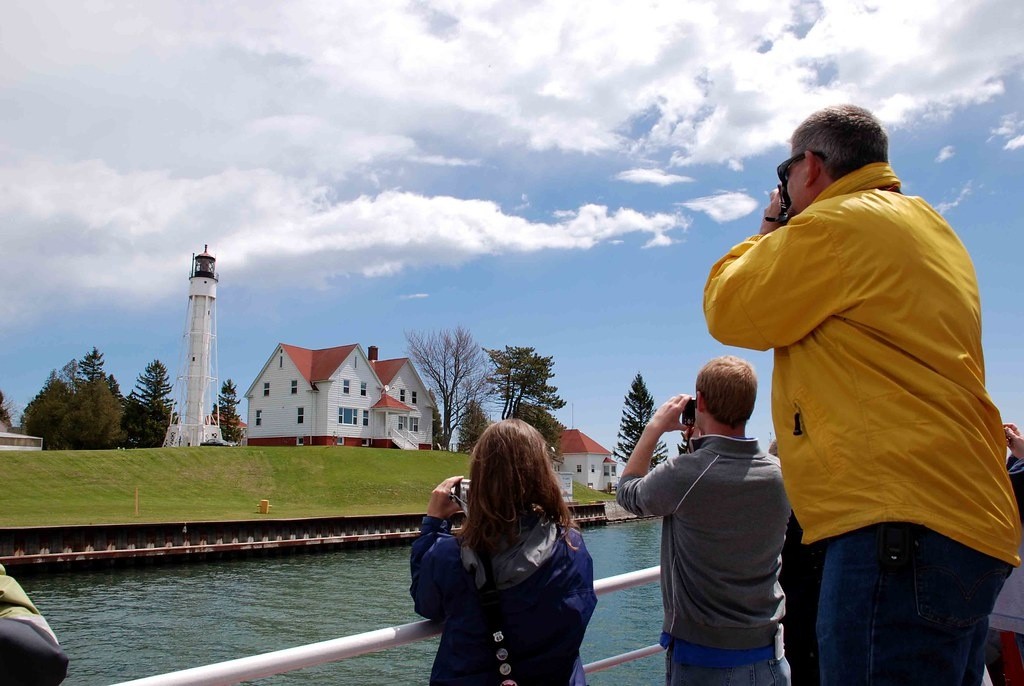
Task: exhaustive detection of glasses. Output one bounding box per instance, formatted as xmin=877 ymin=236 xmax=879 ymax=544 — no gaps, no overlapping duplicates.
xmin=777 ymin=150 xmax=826 ymax=185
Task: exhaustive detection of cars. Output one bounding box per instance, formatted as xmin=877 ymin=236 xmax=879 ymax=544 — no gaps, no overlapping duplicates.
xmin=200 ymin=438 xmax=234 ymax=447
xmin=236 ymin=442 xmax=238 ymax=446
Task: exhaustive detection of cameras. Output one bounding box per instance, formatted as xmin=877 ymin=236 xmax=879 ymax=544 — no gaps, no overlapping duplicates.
xmin=682 ymin=398 xmax=697 ymax=427
xmin=451 ymin=479 xmax=471 ymax=508
xmin=777 ymin=181 xmax=792 ymax=209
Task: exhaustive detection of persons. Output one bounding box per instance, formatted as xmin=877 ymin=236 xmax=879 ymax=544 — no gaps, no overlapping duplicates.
xmin=701 ymin=105 xmax=1024 ymax=686
xmin=410 ymin=417 xmax=598 ymax=686
xmin=767 ymin=423 xmax=1024 ymax=686
xmin=615 ymin=355 xmax=792 ymax=686
